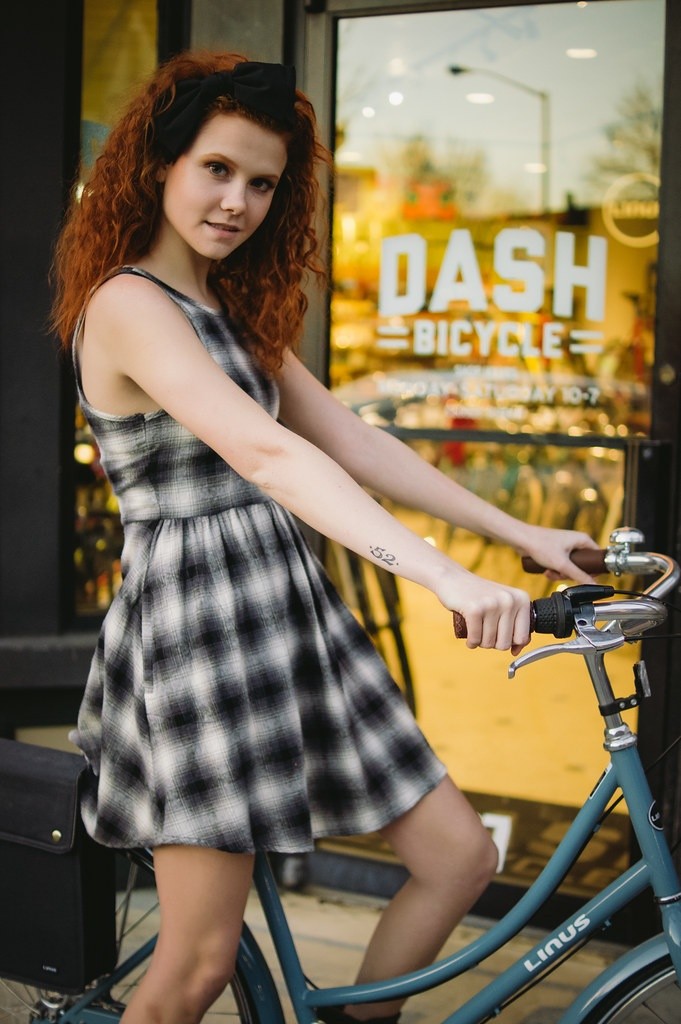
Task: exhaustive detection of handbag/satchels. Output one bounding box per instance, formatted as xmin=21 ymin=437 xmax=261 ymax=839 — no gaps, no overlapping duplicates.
xmin=1 ymin=738 xmax=122 ymax=992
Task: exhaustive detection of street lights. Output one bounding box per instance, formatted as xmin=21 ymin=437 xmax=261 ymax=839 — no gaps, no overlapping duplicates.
xmin=445 ymin=60 xmax=554 ymax=218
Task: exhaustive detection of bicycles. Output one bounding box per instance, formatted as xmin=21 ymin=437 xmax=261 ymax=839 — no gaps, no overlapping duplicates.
xmin=1 ymin=524 xmax=680 ymax=1024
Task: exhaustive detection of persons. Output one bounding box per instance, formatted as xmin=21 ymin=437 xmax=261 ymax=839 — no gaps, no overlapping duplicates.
xmin=49 ymin=50 xmax=598 ymax=1024
